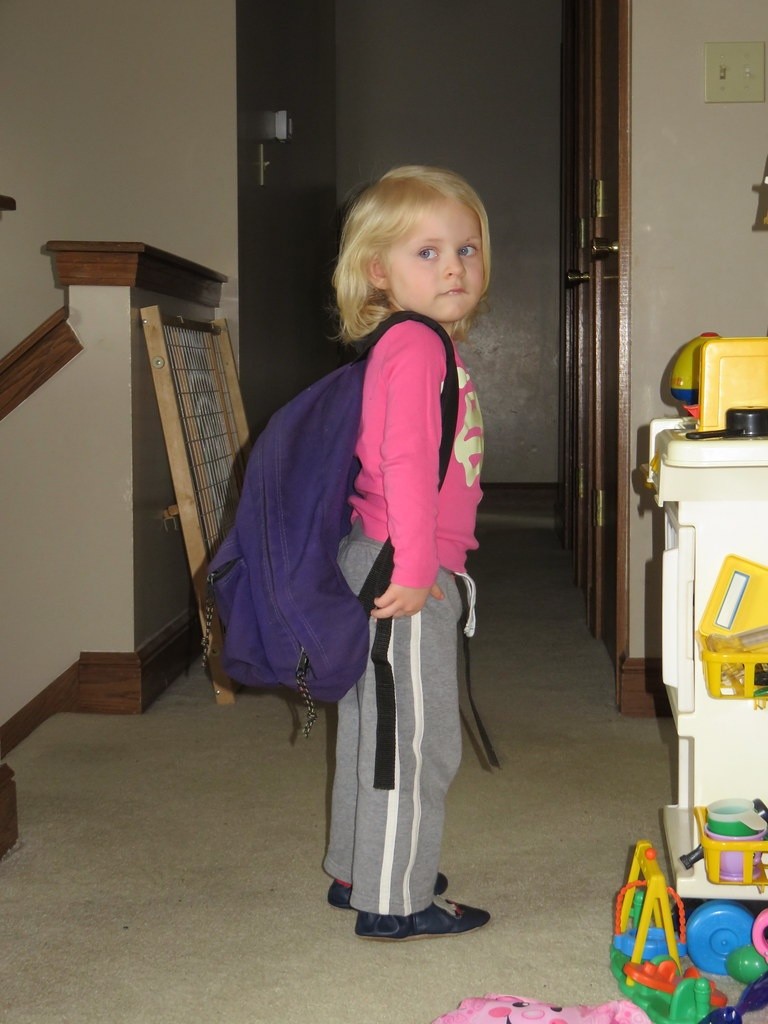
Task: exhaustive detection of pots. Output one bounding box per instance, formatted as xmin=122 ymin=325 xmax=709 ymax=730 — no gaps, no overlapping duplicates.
xmin=685 ymin=405 xmax=768 ymax=440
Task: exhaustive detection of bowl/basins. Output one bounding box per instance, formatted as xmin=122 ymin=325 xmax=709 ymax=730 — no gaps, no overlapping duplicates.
xmin=705 ymin=822 xmax=766 ymax=882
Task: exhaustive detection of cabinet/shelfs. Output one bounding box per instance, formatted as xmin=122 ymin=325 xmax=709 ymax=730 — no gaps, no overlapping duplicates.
xmin=652 ymin=433 xmax=768 ymax=904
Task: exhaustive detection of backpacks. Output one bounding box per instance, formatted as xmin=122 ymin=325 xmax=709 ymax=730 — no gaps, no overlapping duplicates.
xmin=200 ymin=311 xmax=458 ymax=703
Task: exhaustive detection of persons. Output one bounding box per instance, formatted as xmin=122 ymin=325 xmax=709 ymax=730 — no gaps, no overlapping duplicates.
xmin=321 ymin=164 xmax=491 ymax=939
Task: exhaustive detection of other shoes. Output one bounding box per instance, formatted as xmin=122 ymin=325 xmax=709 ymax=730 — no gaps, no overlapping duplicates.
xmin=353 ymin=894 xmax=492 ymax=942
xmin=327 ymin=871 xmax=449 ymax=908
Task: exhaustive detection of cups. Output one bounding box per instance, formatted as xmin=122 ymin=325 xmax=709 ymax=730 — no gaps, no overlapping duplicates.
xmin=706 ymin=797 xmax=766 ymax=836
